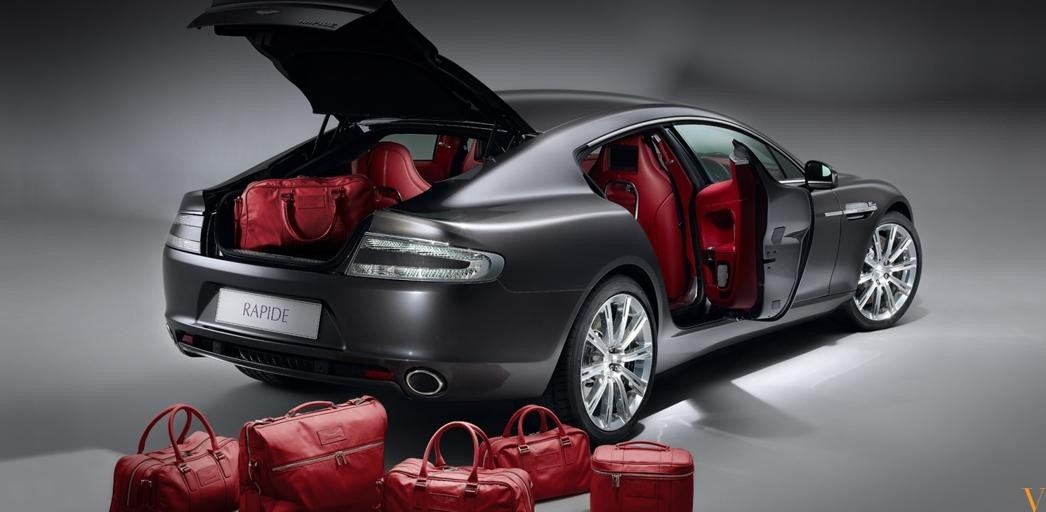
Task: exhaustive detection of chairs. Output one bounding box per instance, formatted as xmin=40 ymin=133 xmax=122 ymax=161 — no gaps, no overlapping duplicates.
xmin=433 ymin=136 xmax=485 ymax=178
xmin=587 ymin=133 xmax=691 ymax=300
xmin=367 ymin=141 xmax=432 ymax=208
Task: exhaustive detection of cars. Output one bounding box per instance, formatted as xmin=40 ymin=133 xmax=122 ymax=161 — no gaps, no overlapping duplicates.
xmin=162 ymin=1 xmax=921 ymax=448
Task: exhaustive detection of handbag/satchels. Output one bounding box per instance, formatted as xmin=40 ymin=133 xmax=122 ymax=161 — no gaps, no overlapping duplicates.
xmin=234 ymin=174 xmax=376 ymax=249
xmin=109 ymin=398 xmax=693 ymax=510
